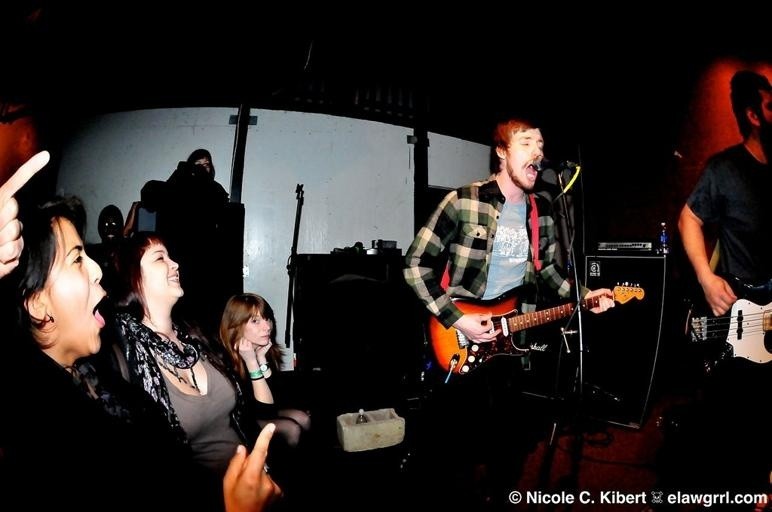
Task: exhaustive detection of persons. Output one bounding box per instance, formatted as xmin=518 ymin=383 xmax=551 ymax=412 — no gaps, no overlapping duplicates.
xmin=668 ymin=60 xmax=771 ymax=511
xmin=186 ymin=148 xmax=215 ymax=180
xmin=214 ymin=293 xmax=333 ymax=511
xmin=0 ymin=194 xmax=290 ymax=512
xmin=91 ymin=222 xmax=297 ymax=511
xmin=1 ymin=147 xmax=56 ymax=275
xmin=388 ymin=110 xmax=615 ymax=511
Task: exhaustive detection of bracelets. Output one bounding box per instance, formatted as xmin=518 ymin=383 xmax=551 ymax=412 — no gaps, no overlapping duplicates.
xmin=248 ymin=369 xmax=265 ymax=382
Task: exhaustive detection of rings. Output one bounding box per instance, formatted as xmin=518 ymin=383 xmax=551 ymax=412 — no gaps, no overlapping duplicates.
xmin=255 ymin=465 xmax=271 ymax=474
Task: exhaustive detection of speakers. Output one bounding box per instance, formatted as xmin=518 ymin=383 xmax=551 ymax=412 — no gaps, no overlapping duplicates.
xmin=583 ymin=252 xmax=669 ymax=434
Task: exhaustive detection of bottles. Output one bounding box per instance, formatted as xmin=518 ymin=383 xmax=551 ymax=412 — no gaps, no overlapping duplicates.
xmin=356 ymin=408 xmax=368 ymax=424
xmin=657 ymin=222 xmax=670 ymax=255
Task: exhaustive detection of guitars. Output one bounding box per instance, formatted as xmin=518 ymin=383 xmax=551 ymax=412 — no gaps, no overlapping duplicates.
xmin=431 ymin=281 xmax=644 ymax=376
xmin=684 ymin=276 xmax=772 ymax=371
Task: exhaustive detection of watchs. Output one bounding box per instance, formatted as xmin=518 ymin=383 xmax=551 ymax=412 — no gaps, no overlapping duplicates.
xmin=259 ymin=362 xmax=270 ymax=373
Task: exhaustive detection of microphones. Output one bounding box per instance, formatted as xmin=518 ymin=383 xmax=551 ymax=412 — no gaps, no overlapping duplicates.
xmin=532 ymin=154 xmax=572 ymax=177
